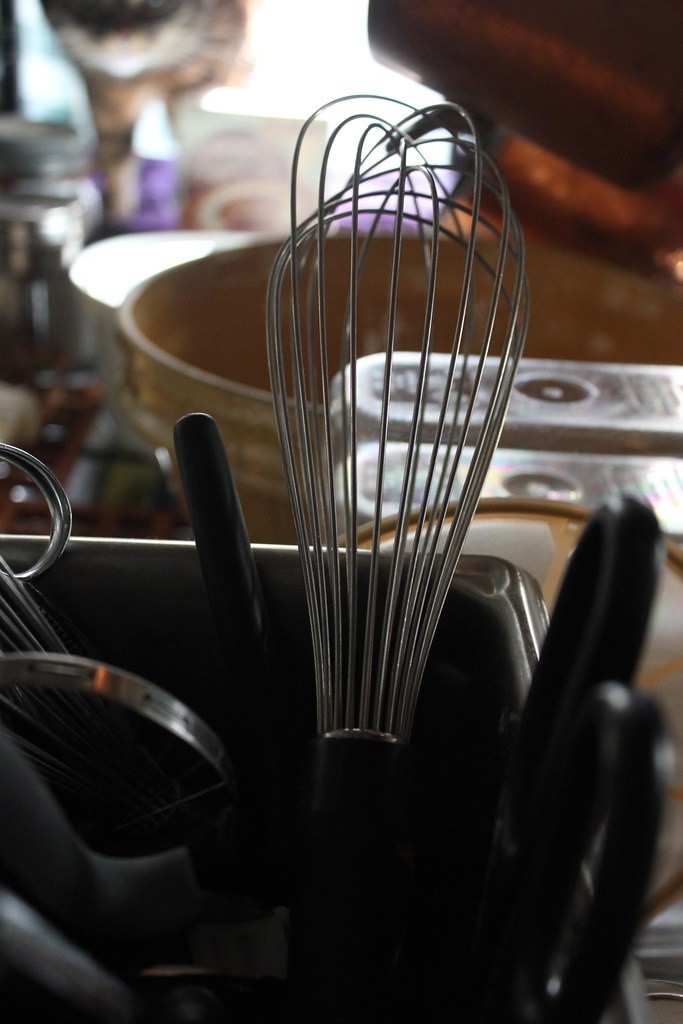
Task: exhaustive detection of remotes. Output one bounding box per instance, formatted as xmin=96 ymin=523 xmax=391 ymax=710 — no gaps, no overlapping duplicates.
xmin=325 ymin=350 xmax=683 ymax=455
xmin=329 ymin=440 xmax=683 ymax=540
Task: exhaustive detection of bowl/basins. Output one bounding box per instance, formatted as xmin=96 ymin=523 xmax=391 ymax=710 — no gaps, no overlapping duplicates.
xmin=120 ymin=234 xmax=683 ymax=552
xmin=68 ymin=228 xmax=272 ymax=364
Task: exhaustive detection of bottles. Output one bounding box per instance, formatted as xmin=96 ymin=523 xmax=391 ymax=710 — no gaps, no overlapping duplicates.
xmin=0 ymin=111 xmax=109 ymax=388
